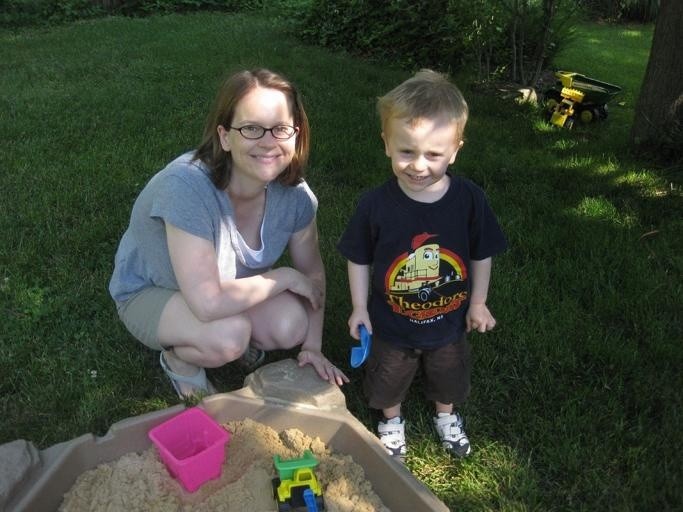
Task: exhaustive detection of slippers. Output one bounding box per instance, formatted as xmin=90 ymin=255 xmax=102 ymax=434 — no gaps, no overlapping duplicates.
xmin=234 ymin=344 xmax=266 ymax=374
xmin=159 ymin=350 xmax=217 ymax=402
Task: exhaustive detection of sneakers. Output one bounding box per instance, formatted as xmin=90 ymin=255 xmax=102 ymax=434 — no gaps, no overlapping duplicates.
xmin=377 ymin=416 xmax=407 ymax=463
xmin=432 ymin=409 xmax=473 ymax=458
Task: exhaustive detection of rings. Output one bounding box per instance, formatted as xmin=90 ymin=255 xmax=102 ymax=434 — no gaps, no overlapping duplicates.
xmin=330 ymin=365 xmax=335 ymax=369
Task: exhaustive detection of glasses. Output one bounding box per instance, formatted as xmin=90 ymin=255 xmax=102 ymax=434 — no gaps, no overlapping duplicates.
xmin=225 ymin=125 xmax=300 ymax=141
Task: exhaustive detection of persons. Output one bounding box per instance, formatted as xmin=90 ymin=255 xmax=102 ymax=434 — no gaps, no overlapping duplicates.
xmin=107 ymin=66 xmax=352 ymax=404
xmin=341 ymin=66 xmax=499 ymax=460
